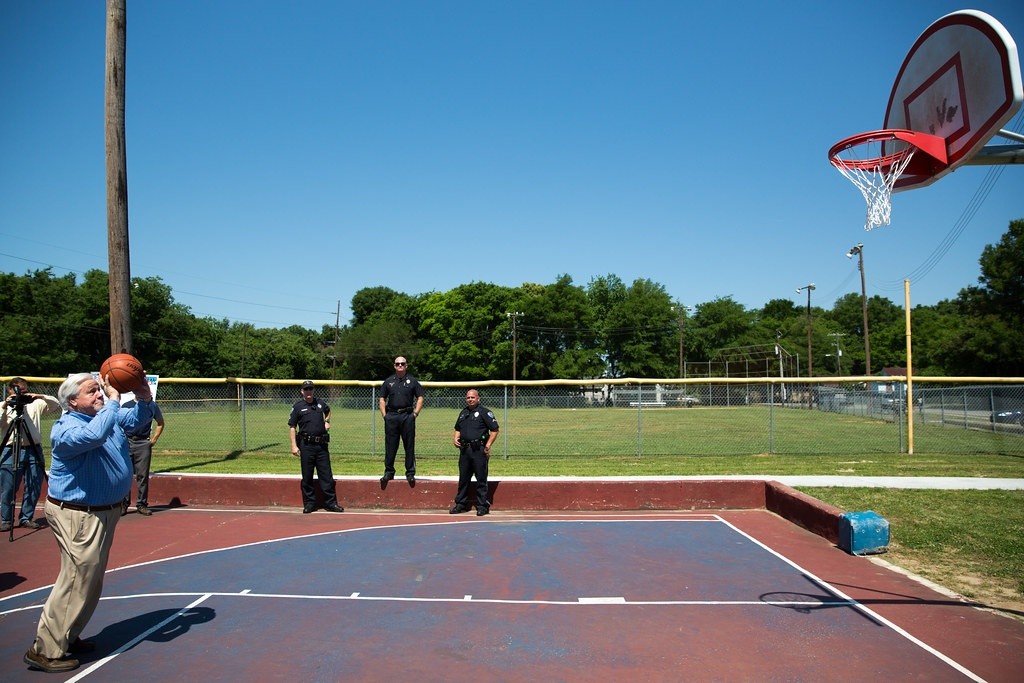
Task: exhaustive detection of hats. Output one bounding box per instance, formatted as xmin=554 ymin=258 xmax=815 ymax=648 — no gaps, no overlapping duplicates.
xmin=302 ymin=381 xmax=314 ymax=388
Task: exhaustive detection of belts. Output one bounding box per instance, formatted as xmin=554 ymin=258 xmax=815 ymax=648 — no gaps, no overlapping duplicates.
xmin=8 ymin=444 xmax=41 ymax=449
xmin=47 ymin=496 xmax=122 ymax=513
xmin=389 ymin=407 xmax=414 ymax=413
xmin=298 ymin=432 xmax=323 ymax=444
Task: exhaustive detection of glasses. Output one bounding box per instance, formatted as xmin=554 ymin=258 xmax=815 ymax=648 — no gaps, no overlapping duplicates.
xmin=303 ymin=386 xmax=314 ymax=391
xmin=396 ymin=362 xmax=406 ymax=366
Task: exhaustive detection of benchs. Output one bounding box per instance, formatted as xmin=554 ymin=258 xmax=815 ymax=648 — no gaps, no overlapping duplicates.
xmin=629 ymin=401 xmax=667 ymax=408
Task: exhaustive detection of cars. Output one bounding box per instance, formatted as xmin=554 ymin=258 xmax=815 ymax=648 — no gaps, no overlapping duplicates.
xmin=677 ymin=395 xmax=699 ymax=405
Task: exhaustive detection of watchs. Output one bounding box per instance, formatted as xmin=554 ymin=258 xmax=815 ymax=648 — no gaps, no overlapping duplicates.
xmin=325 ymin=418 xmax=330 ymax=423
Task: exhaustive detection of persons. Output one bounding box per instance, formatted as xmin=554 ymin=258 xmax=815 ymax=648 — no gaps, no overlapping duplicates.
xmin=377 ymin=357 xmax=424 ymax=482
xmin=24 ymin=372 xmax=156 ymax=673
xmin=0 ymin=377 xmax=165 ymax=530
xmin=449 ymin=389 xmax=500 ymax=516
xmin=288 ymin=381 xmax=344 ymax=513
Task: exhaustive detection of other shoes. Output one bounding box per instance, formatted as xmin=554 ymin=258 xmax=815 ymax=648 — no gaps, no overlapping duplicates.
xmin=450 ymin=503 xmax=471 ymax=514
xmin=136 ymin=505 xmax=152 ymax=515
xmin=19 ymin=519 xmax=39 ymax=529
xmin=477 ymin=507 xmax=490 ymax=515
xmin=120 ymin=506 xmax=127 ymax=516
xmin=380 ymin=474 xmax=394 ymax=481
xmin=407 ymin=475 xmax=415 ymax=482
xmin=1 ymin=523 xmax=15 ymax=532
xmin=23 ymin=643 xmax=80 ymax=673
xmin=326 ymin=504 xmax=344 ymax=512
xmin=66 ymin=637 xmax=85 ymax=654
xmin=303 ymin=507 xmax=318 ymax=513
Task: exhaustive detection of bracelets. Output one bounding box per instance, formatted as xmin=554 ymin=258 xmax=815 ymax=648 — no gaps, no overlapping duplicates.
xmin=412 ymin=411 xmax=418 ymax=417
xmin=137 ymin=393 xmax=153 ymax=400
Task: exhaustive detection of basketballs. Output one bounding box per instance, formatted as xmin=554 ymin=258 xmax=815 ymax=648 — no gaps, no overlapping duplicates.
xmin=100 ymin=353 xmax=143 ymax=394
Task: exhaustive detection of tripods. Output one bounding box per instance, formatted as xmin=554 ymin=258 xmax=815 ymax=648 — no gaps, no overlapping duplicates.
xmin=0 ymin=404 xmax=49 ymax=542
